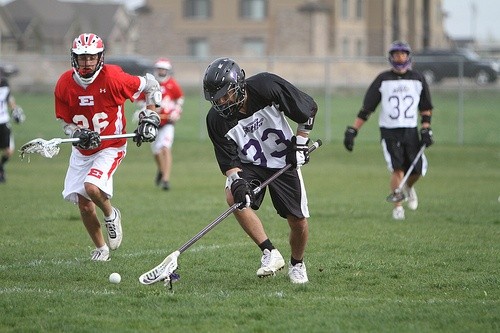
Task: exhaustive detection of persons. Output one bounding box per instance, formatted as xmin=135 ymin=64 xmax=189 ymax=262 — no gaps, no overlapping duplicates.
xmin=132 ymin=56 xmax=184 ymax=190
xmin=0 ymin=65 xmax=25 ymax=183
xmin=344 ymin=40 xmax=434 ymax=220
xmin=202 ymin=57 xmax=319 ymax=284
xmin=54 ymin=33 xmax=162 ymax=261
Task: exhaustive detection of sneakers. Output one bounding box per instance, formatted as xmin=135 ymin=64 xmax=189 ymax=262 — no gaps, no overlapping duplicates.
xmin=256 ymin=249 xmax=285 ymax=278
xmin=287 ymin=259 xmax=308 ymax=284
xmin=88 ymin=242 xmax=109 ymax=261
xmin=103 ymin=206 xmax=122 ymax=250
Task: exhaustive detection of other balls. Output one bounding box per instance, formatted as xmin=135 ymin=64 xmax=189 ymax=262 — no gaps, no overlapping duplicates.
xmin=109 ymin=273 xmax=122 ymax=283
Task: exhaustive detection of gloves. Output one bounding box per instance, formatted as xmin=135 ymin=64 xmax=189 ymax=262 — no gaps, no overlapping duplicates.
xmin=231 ymin=178 xmax=256 ymax=211
xmin=133 ymin=110 xmax=161 ymax=147
xmin=421 ymin=128 xmax=431 ymax=146
xmin=71 ymin=127 xmax=102 ymax=150
xmin=169 ymin=106 xmax=181 ymax=123
xmin=344 ymin=126 xmax=357 ymax=151
xmin=286 ymin=136 xmax=310 ymax=169
xmin=11 ymin=107 xmax=24 ymax=124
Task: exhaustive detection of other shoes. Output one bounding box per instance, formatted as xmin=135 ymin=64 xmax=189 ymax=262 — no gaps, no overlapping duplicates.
xmin=162 ymin=182 xmax=169 ymax=190
xmin=403 ymin=186 xmax=418 ymax=211
xmin=0 ymin=170 xmax=5 ymax=182
xmin=392 ymin=206 xmax=405 ymax=221
xmin=155 ymin=172 xmax=161 ymax=185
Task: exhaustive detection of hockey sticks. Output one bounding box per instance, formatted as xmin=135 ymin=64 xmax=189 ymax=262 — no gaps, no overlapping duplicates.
xmin=386 ymin=144 xmax=428 ymax=204
xmin=20 ymin=131 xmax=139 ymax=160
xmin=139 ymin=139 xmax=324 ymax=286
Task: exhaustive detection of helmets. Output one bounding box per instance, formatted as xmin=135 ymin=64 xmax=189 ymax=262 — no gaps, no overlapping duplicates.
xmin=202 ymin=58 xmax=246 ymax=119
xmin=154 ymin=58 xmax=172 ymax=84
xmin=70 ymin=33 xmax=105 ymax=85
xmin=0 ymin=65 xmax=18 ymax=79
xmin=388 ymin=40 xmax=412 ymax=73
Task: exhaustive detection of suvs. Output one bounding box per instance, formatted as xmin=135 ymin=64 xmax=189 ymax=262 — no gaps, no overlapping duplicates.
xmin=105 ymin=53 xmax=174 ymax=77
xmin=411 ymin=48 xmax=500 ymax=85
